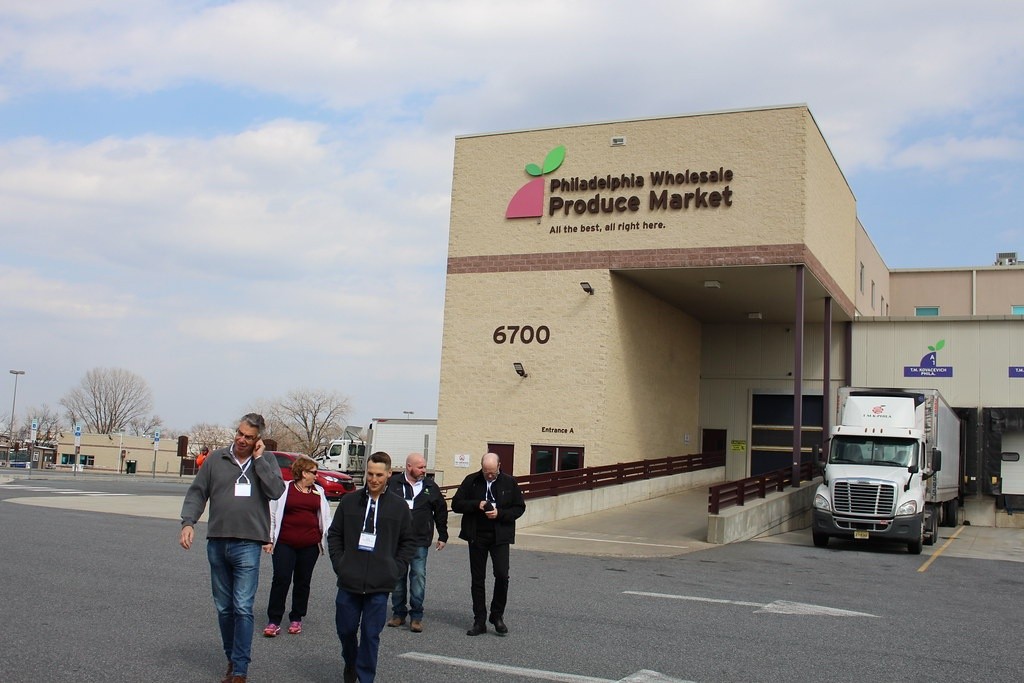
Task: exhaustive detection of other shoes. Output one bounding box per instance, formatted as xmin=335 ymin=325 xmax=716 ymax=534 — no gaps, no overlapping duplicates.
xmin=388 ymin=617 xmax=406 ymax=626
xmin=264 ymin=623 xmax=281 ymax=636
xmin=232 ymin=677 xmax=246 ymax=683
xmin=467 ymin=628 xmax=487 ymax=636
xmin=221 ymin=663 xmax=232 ymax=683
xmin=489 ymin=618 xmax=508 ymax=634
xmin=288 ymin=621 xmax=302 ymax=633
xmin=410 ymin=620 xmax=422 ymax=632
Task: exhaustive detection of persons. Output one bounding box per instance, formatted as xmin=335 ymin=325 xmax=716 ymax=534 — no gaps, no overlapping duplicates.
xmin=451 ymin=452 xmax=526 ymax=636
xmin=386 ymin=452 xmax=448 ymax=632
xmin=196 ymin=447 xmax=212 ymax=469
xmin=327 ymin=451 xmax=418 ymax=683
xmin=263 ymin=456 xmax=333 ymax=637
xmin=180 ymin=414 xmax=286 ymax=683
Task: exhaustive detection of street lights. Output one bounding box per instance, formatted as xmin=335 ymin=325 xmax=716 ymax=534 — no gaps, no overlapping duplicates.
xmin=403 ymin=411 xmax=414 ymax=419
xmin=5 ymin=370 xmax=25 ymax=469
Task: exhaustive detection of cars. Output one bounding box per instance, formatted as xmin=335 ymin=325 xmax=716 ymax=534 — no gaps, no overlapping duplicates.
xmin=267 ymin=451 xmax=356 ymax=501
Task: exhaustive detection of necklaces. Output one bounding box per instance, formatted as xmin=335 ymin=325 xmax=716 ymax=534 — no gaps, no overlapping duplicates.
xmin=294 ymin=482 xmax=311 ymax=493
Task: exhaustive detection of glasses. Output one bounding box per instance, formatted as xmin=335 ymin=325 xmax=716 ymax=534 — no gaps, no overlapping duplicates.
xmin=308 ymin=470 xmax=317 ymax=475
xmin=482 ymin=464 xmax=499 ymax=475
xmin=236 ymin=428 xmax=257 ymax=440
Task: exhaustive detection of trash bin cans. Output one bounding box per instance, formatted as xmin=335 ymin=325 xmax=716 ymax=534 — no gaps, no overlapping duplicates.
xmin=125 ymin=460 xmax=136 ymax=473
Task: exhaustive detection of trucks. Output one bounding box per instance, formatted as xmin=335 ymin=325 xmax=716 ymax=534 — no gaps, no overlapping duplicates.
xmin=314 ymin=418 xmax=437 ymax=486
xmin=808 ymin=386 xmax=960 ymax=556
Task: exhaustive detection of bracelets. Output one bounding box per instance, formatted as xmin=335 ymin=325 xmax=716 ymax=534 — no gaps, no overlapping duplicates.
xmin=255 ymin=455 xmax=262 ymax=460
